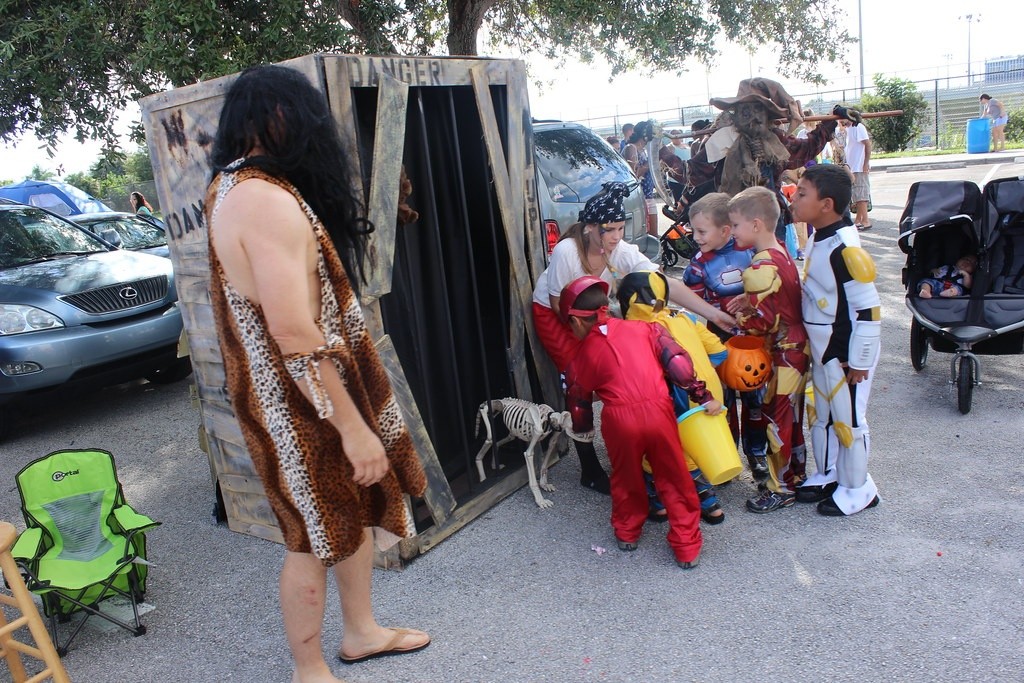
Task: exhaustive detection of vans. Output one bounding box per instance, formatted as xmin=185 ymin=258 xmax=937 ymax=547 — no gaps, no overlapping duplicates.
xmin=0 ymin=175 xmax=115 ymax=218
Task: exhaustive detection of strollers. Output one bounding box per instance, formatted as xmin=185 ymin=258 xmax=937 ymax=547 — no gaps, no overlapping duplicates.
xmin=898 ymin=176 xmax=1023 ymax=416
xmin=659 ymin=204 xmax=702 ymax=268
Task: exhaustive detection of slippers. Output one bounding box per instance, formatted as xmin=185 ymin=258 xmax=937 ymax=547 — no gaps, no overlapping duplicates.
xmin=340 ymin=627 xmax=431 ymax=663
xmin=855 ymin=222 xmax=872 ymax=230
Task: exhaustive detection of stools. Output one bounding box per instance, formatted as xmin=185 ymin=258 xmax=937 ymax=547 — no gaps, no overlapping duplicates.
xmin=0 ymin=520 xmax=71 ymax=683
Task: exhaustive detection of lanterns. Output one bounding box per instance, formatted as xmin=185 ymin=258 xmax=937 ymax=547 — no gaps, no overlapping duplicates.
xmin=717 ymin=338 xmax=772 ymax=392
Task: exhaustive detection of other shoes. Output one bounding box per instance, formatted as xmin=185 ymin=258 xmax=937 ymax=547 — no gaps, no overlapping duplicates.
xmin=578 ymin=472 xmax=611 ymax=495
xmin=677 ymin=545 xmax=702 ymax=569
xmin=616 ymin=537 xmax=637 ymax=552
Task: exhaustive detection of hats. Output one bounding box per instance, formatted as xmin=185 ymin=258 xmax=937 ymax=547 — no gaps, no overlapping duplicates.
xmin=578 ymin=179 xmax=630 ymax=224
xmin=709 ymin=78 xmax=806 ymax=144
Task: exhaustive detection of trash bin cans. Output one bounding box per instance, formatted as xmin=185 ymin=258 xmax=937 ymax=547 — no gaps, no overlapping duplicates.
xmin=966 ymin=117 xmax=991 ymax=154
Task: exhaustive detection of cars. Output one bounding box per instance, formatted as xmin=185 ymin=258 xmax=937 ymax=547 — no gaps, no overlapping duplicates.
xmin=68 ymin=213 xmax=171 ymax=263
xmin=0 ymin=194 xmax=193 ymax=416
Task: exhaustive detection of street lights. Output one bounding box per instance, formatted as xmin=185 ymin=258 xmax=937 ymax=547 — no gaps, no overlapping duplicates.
xmin=957 ymin=12 xmax=981 ymax=86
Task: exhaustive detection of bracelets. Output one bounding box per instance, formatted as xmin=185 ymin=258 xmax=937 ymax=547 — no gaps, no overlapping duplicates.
xmin=999 ymin=116 xmax=1002 ymax=118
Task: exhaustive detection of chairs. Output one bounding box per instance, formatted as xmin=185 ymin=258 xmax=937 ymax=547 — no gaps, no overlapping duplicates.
xmin=10 ymin=448 xmax=162 ymax=657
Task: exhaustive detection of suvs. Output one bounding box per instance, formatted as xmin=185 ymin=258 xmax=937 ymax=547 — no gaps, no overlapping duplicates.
xmin=530 ymin=118 xmax=663 ymax=267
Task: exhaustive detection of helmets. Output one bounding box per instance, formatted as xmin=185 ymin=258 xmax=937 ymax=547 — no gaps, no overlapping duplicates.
xmin=559 ymin=275 xmax=609 ymax=324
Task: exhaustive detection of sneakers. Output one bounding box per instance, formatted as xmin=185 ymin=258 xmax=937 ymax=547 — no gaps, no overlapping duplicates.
xmin=797 ymin=480 xmax=839 ymax=501
xmin=819 ymin=495 xmax=879 ymax=517
xmin=747 ymin=454 xmax=769 ymax=480
xmin=746 ymin=487 xmax=795 ymax=513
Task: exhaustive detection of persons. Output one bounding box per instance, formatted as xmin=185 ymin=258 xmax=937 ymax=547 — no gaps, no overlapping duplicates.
xmin=205 ymin=65 xmax=430 ymax=683
xmin=676 ymin=77 xmax=862 ymax=244
xmin=683 ymin=191 xmax=770 ymax=479
xmin=727 ymin=186 xmax=811 ymax=514
xmin=780 ymin=108 xmax=872 ymax=260
xmin=917 ymin=253 xmax=977 ymax=298
xmin=617 ymin=271 xmax=728 ymax=525
xmin=606 ymin=119 xmax=707 ymax=238
xmin=131 ymin=192 xmax=153 ymax=216
xmin=532 ymin=183 xmax=740 ymax=493
xmin=790 ymin=163 xmax=883 ymax=517
xmin=559 ymin=276 xmax=723 ymax=570
xmin=980 ymin=94 xmax=1007 ymax=152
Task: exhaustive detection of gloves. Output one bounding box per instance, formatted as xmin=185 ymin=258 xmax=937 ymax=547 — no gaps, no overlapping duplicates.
xmin=832 ymin=105 xmax=862 ymax=126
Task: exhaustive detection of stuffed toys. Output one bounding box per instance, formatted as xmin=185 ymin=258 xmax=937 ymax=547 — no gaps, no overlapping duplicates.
xmin=397 ymin=165 xmax=418 ymax=225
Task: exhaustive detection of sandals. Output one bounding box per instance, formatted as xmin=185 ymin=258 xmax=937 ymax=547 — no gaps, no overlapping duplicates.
xmin=647 ymin=505 xmax=668 ymax=523
xmin=700 ymin=502 xmax=725 ymax=525
